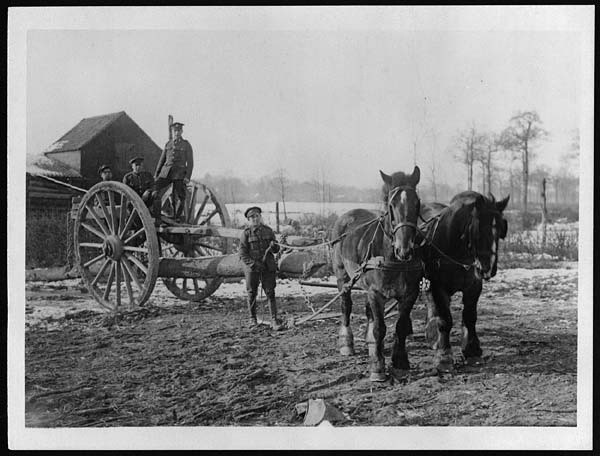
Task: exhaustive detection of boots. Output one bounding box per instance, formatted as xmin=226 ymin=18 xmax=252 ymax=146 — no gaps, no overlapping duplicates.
xmin=264 ymin=289 xmax=283 ymax=330
xmin=175 ymin=197 xmax=185 ymax=222
xmin=152 ymin=200 xmax=161 ymax=227
xmin=247 ymin=289 xmax=258 ymax=328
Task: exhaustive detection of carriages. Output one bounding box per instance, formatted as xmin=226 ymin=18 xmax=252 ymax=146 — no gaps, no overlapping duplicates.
xmin=73 ymin=165 xmax=510 ymax=381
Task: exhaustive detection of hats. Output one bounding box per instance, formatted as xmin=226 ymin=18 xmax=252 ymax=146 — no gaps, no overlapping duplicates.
xmin=170 ymin=123 xmax=184 ymax=130
xmin=244 ymin=207 xmax=262 ymax=218
xmin=129 ymin=158 xmax=144 ymax=165
xmin=97 ymin=165 xmax=112 ymax=175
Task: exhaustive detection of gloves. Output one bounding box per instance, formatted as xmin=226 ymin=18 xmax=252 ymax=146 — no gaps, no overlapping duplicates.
xmin=253 ymin=262 xmax=266 ymax=273
xmin=270 ymin=241 xmax=277 ymax=250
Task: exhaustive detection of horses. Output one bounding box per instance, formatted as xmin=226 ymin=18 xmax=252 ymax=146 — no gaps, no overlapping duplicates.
xmin=332 ymin=166 xmax=424 ymax=383
xmin=418 ymin=190 xmax=510 ymax=361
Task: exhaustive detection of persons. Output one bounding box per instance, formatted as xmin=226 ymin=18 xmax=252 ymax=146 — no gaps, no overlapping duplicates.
xmin=239 ymin=207 xmax=284 ymax=331
xmin=122 ymin=156 xmax=153 ymax=196
xmin=154 ymin=123 xmax=194 ymax=221
xmin=97 ymin=164 xmax=113 ymax=181
